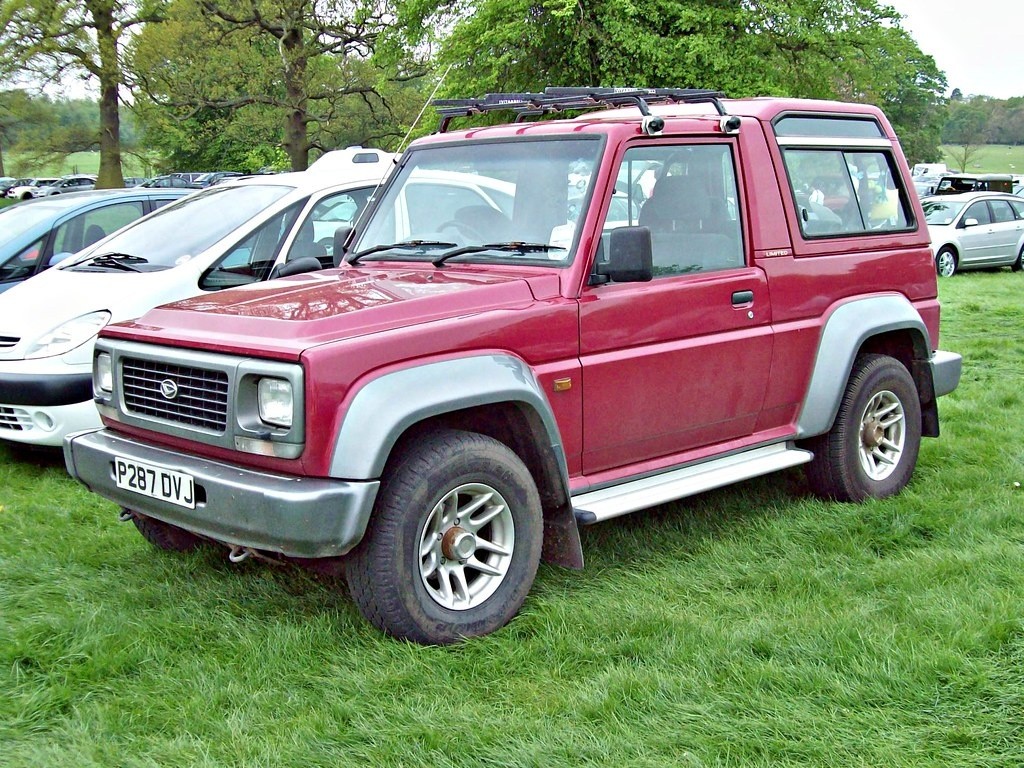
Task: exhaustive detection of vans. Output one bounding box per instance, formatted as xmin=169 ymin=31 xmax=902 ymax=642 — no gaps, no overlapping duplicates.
xmin=908 ymin=163 xmax=948 ymax=175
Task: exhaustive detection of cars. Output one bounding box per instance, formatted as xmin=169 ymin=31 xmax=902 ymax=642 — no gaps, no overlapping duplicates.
xmin=1 ymin=188 xmax=200 ymax=291
xmin=0 ymin=170 xmax=234 ymax=199
xmin=0 ymin=152 xmax=517 ymax=448
xmin=918 ymin=193 xmax=1023 ymax=277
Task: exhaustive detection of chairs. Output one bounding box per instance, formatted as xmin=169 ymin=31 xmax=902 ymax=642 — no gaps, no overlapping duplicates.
xmin=643 ymin=171 xmax=725 ymax=276
xmin=284 ymin=208 xmax=328 ymax=260
xmin=83 ymin=224 xmax=106 ymax=246
xmin=636 ymin=195 xmax=671 ymax=232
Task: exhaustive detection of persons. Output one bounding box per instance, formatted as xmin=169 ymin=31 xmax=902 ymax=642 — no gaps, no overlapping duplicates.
xmin=810 ymin=182 xmax=824 ymax=206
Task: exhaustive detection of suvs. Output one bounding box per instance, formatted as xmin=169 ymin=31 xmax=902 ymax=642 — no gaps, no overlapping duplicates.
xmin=59 ymin=87 xmax=961 ymax=648
xmin=794 ymin=171 xmax=857 ymax=214
xmin=930 ymin=173 xmax=1014 ymax=198
